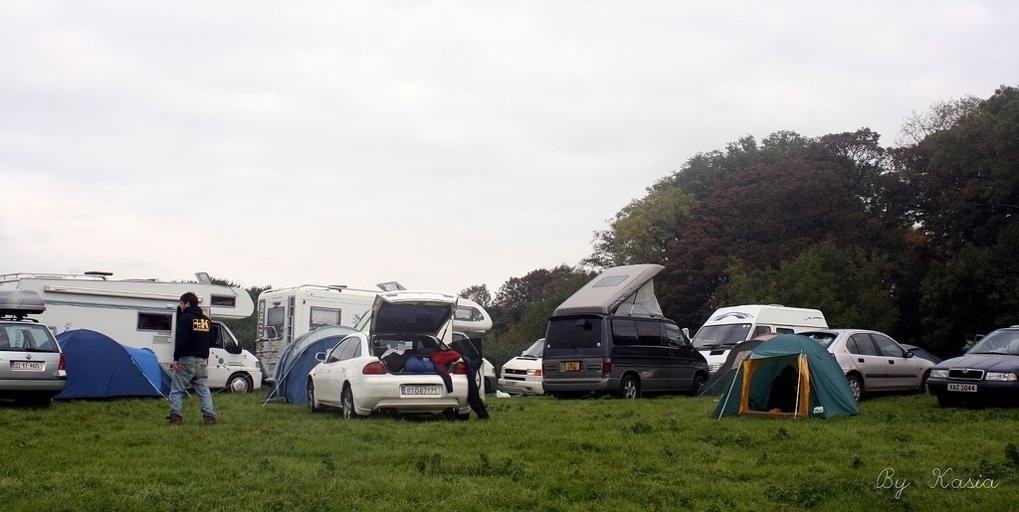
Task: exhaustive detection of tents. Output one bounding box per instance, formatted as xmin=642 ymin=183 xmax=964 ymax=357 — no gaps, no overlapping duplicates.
xmin=40 ymin=329 xmax=191 ymax=401
xmin=272 ymin=323 xmax=360 ymax=405
xmin=712 ymin=333 xmax=861 ymax=419
xmin=696 ymin=332 xmax=781 ymax=398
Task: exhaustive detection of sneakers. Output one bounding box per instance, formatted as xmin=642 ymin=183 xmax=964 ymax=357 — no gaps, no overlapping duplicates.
xmin=203 ymin=416 xmax=215 ymax=425
xmin=166 ymin=414 xmax=182 ymax=423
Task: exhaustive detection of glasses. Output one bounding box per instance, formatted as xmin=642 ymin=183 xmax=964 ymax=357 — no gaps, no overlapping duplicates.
xmin=180 ymin=301 xmax=184 ymax=305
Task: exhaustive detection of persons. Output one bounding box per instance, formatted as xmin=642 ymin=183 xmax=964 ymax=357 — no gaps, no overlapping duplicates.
xmin=421 ymin=346 xmax=490 ymax=420
xmin=165 ymin=291 xmax=217 ymax=425
xmin=766 ymin=364 xmax=798 ymax=412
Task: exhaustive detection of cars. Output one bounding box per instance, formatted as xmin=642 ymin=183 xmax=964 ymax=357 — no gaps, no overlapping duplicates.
xmin=1 ymin=264 xmax=1018 ymax=420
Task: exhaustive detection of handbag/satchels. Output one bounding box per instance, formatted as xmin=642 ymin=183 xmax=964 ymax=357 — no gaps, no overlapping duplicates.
xmin=383 ymin=347 xmax=436 ymax=375
